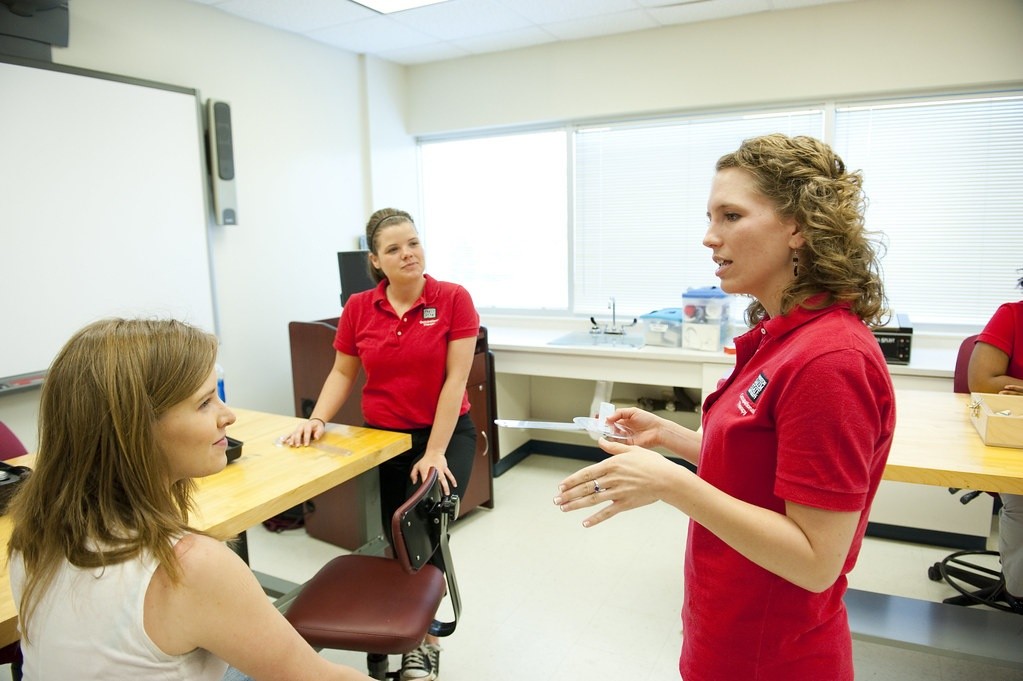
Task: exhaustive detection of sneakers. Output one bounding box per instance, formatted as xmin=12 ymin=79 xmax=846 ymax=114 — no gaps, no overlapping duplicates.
xmin=402 ymin=646 xmax=432 ymax=677
xmin=425 ymin=643 xmax=441 ymax=680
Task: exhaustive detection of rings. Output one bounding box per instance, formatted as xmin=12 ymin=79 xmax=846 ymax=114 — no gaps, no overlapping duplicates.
xmin=593 ymin=480 xmax=600 ymax=492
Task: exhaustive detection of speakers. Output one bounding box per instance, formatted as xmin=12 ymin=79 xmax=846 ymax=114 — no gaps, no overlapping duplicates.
xmin=206 ymin=96 xmax=238 ymax=226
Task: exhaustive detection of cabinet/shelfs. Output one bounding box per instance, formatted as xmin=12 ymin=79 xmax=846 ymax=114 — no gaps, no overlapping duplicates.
xmin=289 ymin=317 xmax=494 ymax=554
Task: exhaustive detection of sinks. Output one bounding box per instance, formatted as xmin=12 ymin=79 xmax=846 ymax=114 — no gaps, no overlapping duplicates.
xmin=547 ymin=328 xmax=646 ymax=350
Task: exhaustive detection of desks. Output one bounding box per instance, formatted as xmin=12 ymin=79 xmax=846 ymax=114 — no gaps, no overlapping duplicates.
xmin=819 ymin=387 xmax=1023 ymax=681
xmin=0 ymin=400 xmax=411 ymax=681
xmin=480 ymin=330 xmax=969 ymax=477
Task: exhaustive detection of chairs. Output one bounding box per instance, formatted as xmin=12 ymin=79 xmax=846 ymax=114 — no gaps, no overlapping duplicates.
xmin=927 ymin=334 xmax=1023 ymax=614
xmin=285 ymin=466 xmax=462 ymax=681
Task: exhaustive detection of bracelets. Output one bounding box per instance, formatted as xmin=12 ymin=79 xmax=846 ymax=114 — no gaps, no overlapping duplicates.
xmin=310 ymin=418 xmax=325 ymax=426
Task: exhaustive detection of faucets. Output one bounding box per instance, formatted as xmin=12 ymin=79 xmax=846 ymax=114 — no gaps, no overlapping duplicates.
xmin=604 ymin=295 xmax=638 ymax=335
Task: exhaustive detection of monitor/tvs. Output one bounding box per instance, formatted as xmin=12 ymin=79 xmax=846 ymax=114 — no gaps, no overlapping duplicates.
xmin=338 ymin=250 xmax=379 ymax=307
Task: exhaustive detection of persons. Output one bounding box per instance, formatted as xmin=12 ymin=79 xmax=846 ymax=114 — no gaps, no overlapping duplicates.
xmin=283 ymin=209 xmax=480 ymax=681
xmin=553 ymin=131 xmax=896 ymax=681
xmin=5 ymin=318 xmax=380 ymax=681
xmin=967 ymin=268 xmax=1023 ymax=612
